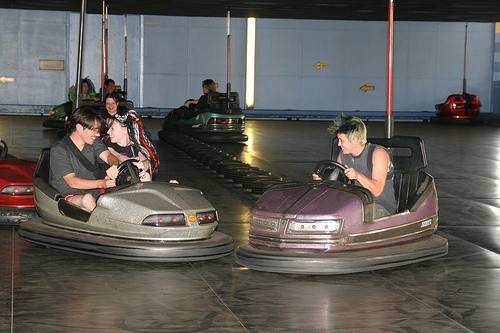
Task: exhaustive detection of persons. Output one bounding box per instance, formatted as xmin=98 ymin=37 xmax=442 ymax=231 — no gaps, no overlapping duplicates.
xmin=183 ymin=78 xmax=227 ymax=119
xmin=99 ymin=93 xmax=128 ymax=139
xmin=95 ymin=79 xmax=124 ymax=103
xmin=48 ymin=106 xmax=119 ymax=213
xmin=99 ymin=107 xmax=158 ymax=184
xmin=72 ymin=79 xmax=100 ymax=101
xmin=311 ymin=112 xmax=397 ymax=221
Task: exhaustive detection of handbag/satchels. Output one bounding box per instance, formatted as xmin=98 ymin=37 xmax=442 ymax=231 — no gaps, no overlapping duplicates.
xmin=94 ymin=164 xmax=108 ymax=179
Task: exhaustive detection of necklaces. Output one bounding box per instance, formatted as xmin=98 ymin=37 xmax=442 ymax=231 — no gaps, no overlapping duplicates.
xmin=125 ymin=139 xmax=131 ymax=146
